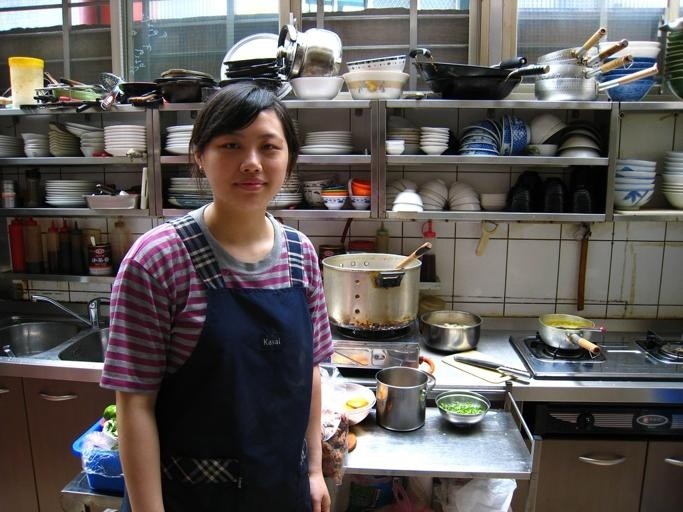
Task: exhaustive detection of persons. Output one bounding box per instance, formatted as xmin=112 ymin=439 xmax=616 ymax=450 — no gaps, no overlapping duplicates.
xmin=99 ymin=82 xmax=332 ymax=511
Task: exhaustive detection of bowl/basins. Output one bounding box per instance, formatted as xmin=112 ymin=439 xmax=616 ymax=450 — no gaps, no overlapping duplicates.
xmin=435 ymin=390 xmax=492 ymax=428
xmin=287 ymin=75 xmax=344 ymax=101
xmin=385 ymin=113 xmax=604 ymax=215
xmin=118 ymin=82 xmax=156 ymax=97
xmin=341 ymin=54 xmax=409 ymax=101
xmin=156 ymin=59 xmax=282 ymax=101
xmin=335 ymin=383 xmax=376 ymax=425
xmin=419 ymin=310 xmax=483 ymax=352
xmin=303 ymin=174 xmax=370 ymax=210
xmin=664 ymin=22 xmax=682 ymax=100
xmin=50 ymin=88 xmax=96 ymax=100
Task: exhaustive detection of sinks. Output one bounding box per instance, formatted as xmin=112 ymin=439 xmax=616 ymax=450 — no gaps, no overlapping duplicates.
xmin=58 ymin=330 xmax=110 ymax=364
xmin=0 ymin=321 xmax=82 ymax=358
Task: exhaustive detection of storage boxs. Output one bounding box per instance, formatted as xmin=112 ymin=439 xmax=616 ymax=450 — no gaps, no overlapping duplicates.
xmin=73 ymin=415 xmax=127 ymax=492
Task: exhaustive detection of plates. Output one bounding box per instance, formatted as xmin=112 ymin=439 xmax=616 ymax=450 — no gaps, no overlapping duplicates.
xmin=41 ymin=178 xmax=93 ymax=207
xmin=289 ymin=118 xmax=353 ymax=155
xmin=163 ymin=123 xmax=194 ymax=154
xmin=166 ymin=176 xmax=213 ymax=209
xmin=0 ymin=119 xmax=146 ymax=159
xmin=263 ymin=172 xmax=302 ymax=208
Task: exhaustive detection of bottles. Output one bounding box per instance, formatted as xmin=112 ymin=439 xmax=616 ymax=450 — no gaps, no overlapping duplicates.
xmin=349 ymin=240 xmax=375 ymax=254
xmin=1 ymin=180 xmax=16 ymax=208
xmin=24 ymin=171 xmax=40 ymax=208
xmin=7 ymin=215 xmax=86 ymax=275
xmin=375 ymin=222 xmax=388 ymax=253
xmin=419 ymin=219 xmax=437 ymax=283
xmin=110 ymin=218 xmax=131 ymax=274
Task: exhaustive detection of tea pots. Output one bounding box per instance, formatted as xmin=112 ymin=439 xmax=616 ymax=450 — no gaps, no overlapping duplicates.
xmin=275 ymin=25 xmax=341 ymax=77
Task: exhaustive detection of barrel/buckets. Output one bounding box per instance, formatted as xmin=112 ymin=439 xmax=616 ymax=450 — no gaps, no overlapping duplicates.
xmin=374 ymin=365 xmax=437 ymax=434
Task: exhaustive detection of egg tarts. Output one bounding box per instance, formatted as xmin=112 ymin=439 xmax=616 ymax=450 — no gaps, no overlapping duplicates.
xmin=345 ymin=396 xmax=369 ymax=409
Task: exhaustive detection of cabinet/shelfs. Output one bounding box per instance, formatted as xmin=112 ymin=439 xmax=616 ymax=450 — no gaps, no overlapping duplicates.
xmin=149 ymin=99 xmax=378 ymax=221
xmin=0 ymin=375 xmax=116 ymax=511
xmin=377 ymin=100 xmax=610 ymax=224
xmin=0 ymin=104 xmax=155 ymax=285
xmin=522 ymin=439 xmax=682 ymax=512
xmin=609 ymin=101 xmax=683 ymax=223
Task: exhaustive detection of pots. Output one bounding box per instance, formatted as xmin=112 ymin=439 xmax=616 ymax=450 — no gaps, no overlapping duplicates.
xmin=320 ymin=252 xmax=422 ymax=331
xmin=410 ymin=28 xmax=664 ymax=101
xmin=614 ymin=147 xmax=682 ymax=211
xmin=537 ymin=314 xmax=602 ymax=355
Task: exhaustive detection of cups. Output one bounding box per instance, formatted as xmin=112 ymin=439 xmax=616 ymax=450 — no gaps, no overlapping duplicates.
xmin=317 ymin=244 xmax=344 ymax=281
xmin=86 ymin=244 xmax=111 ymax=275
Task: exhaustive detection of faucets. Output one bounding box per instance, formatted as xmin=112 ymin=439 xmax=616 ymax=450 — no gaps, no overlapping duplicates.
xmin=29 ymin=293 xmax=102 ymax=330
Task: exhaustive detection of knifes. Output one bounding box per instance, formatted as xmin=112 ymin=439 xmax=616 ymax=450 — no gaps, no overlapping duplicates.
xmin=453 ymin=356 xmax=532 ymax=380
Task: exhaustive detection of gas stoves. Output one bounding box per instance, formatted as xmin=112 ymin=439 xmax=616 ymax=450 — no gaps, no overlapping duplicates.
xmin=326 ymin=322 xmax=419 ymax=372
xmin=508 ymin=330 xmax=683 ymax=440
